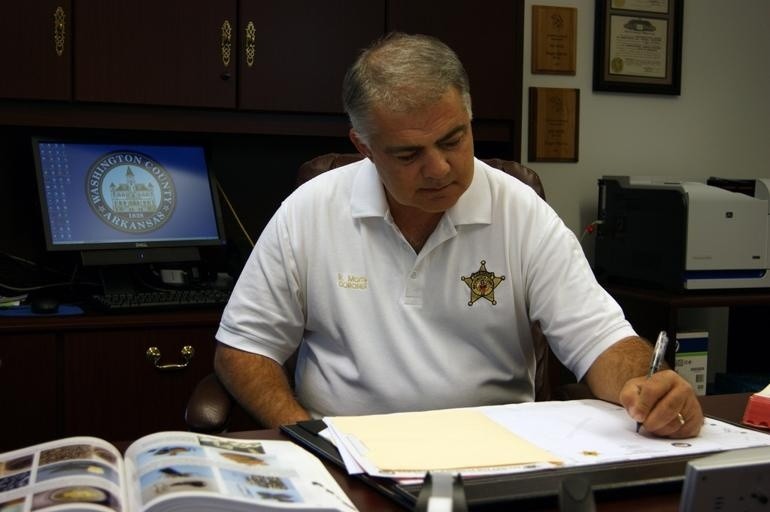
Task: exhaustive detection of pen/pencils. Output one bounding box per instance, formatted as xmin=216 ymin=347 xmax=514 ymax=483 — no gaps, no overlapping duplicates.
xmin=637 ymin=330 xmax=669 ymax=433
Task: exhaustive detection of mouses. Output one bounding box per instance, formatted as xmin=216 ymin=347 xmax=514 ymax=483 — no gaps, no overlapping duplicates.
xmin=31 ymin=291 xmax=61 ymax=314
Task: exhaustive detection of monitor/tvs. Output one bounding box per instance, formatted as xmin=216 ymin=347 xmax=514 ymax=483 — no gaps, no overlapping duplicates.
xmin=29 ymin=133 xmax=227 ymax=269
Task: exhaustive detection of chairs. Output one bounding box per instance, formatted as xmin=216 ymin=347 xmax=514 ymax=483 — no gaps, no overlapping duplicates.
xmin=178 ymin=373 xmax=234 ymax=433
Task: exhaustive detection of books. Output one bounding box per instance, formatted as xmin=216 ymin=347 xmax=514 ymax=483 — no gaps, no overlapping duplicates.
xmin=0 ymin=429 xmax=360 ymax=511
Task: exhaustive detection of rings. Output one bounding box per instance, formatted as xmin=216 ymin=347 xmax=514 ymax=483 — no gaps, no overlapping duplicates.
xmin=677 ymin=413 xmax=685 ymax=425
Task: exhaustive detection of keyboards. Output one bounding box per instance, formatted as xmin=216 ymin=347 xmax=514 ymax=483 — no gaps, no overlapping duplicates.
xmin=93 ymin=285 xmax=234 ymax=311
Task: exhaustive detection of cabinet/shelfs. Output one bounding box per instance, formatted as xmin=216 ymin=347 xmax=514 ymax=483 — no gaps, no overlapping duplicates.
xmin=352 ymin=0 xmax=527 ymax=156
xmin=592 ymin=287 xmax=770 ymax=393
xmin=1 ymin=0 xmax=78 ymax=108
xmin=0 ymin=314 xmax=68 ymax=454
xmin=70 ymin=0 xmax=362 ymax=117
xmin=67 ymin=308 xmax=301 ymax=443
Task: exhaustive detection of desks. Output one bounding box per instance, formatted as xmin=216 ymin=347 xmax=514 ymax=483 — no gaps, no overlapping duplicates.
xmin=205 ymin=395 xmax=769 ymax=511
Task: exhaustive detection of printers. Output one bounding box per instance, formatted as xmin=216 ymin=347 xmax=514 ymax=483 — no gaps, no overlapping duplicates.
xmin=589 ymin=172 xmax=770 ymax=294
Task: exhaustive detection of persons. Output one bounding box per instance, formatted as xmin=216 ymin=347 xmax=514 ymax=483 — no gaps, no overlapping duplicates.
xmin=213 ymin=30 xmax=705 ymax=440
